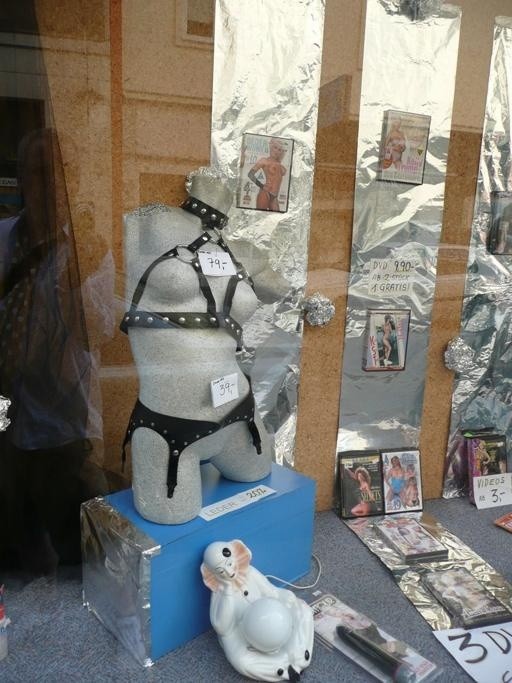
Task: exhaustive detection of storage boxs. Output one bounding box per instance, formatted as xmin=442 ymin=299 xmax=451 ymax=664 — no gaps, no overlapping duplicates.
xmin=81 ymin=463 xmax=317 ymax=668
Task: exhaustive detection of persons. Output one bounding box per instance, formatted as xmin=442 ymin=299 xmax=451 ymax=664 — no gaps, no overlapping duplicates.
xmin=246 ymin=137 xmax=288 ymax=212
xmin=120 ymin=164 xmax=295 ymax=529
xmin=441 ymin=572 xmax=479 ymax=608
xmin=382 ymin=117 xmax=406 ymax=172
xmin=344 ymin=464 xmax=375 ymax=516
xmin=381 ymin=315 xmax=396 ymax=366
xmin=476 ymin=442 xmax=491 ymax=476
xmin=379 ymin=518 xmax=436 ymax=553
xmin=385 ymin=455 xmax=419 ymax=507
xmin=319 ymin=602 xmax=409 ymax=666
